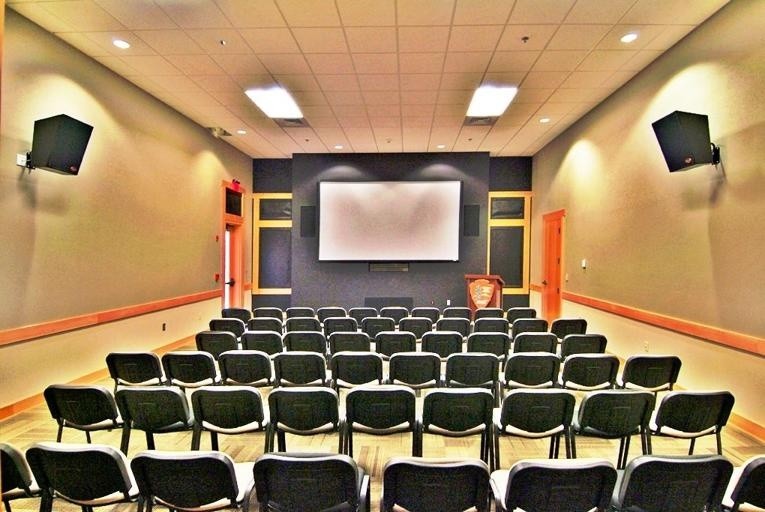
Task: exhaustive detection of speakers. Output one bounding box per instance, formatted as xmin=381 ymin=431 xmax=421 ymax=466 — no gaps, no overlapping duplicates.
xmin=651 ymin=111 xmax=712 ymax=173
xmin=28 ymin=113 xmax=94 ymax=176
